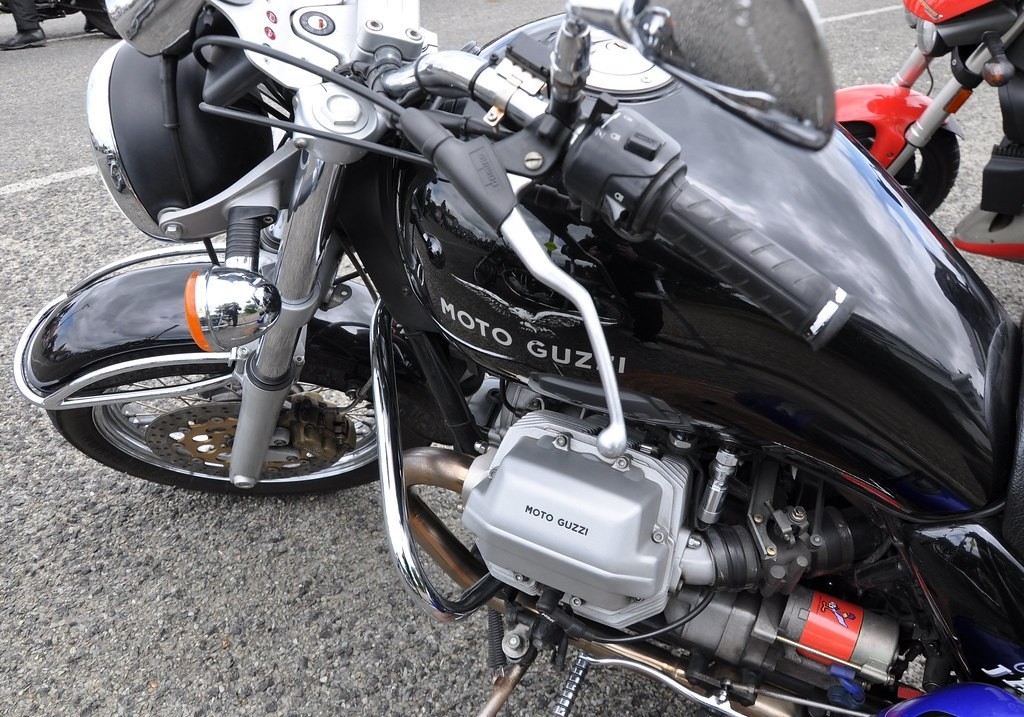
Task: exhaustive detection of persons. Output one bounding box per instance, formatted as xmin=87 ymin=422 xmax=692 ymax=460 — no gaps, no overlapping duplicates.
xmin=0 ymin=0 xmax=101 ymax=52
xmin=228 ymin=302 xmax=242 ymax=327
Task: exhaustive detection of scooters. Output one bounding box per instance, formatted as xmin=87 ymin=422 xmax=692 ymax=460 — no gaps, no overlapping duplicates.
xmin=825 ymin=0 xmax=1024 ymax=270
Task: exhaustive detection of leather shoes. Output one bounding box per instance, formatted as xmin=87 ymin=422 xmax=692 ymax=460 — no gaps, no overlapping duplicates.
xmin=0 ymin=28 xmax=46 ymax=48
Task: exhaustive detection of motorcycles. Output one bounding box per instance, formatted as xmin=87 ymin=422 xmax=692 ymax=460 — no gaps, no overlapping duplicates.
xmin=12 ymin=0 xmax=1023 ymax=717
